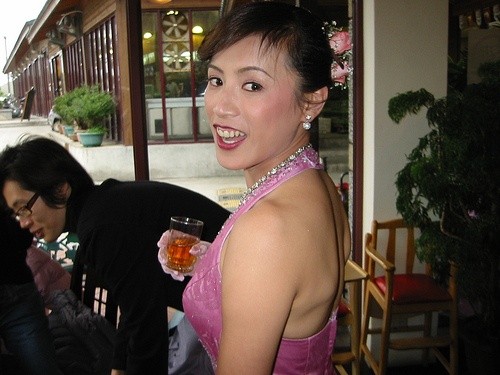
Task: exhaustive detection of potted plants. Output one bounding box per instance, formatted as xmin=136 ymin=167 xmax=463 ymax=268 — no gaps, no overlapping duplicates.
xmin=389 ymin=60 xmax=500 ymax=375
xmin=52 ymin=82 xmax=118 ymax=146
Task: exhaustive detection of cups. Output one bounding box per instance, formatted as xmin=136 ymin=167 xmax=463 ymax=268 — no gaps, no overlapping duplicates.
xmin=166 ymin=217 xmax=204 ymax=273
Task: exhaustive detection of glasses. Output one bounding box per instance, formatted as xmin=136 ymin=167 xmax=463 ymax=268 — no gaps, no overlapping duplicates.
xmin=12 ymin=192 xmax=40 ymax=222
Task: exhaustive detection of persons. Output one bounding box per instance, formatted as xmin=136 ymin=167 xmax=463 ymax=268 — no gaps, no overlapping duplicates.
xmin=0 ymin=138 xmax=231 ymax=375
xmin=158 ymin=0 xmax=351 ymax=375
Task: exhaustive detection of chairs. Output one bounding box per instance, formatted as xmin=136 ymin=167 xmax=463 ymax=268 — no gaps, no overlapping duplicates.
xmin=46 ymin=247 xmax=118 ymax=375
xmin=360 ymin=219 xmax=459 ymax=375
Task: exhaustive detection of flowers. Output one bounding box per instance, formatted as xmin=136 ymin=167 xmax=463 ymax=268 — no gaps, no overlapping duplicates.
xmin=322 ymin=20 xmax=355 ymax=90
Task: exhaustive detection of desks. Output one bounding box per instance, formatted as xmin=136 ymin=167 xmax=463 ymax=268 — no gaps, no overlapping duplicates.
xmin=331 ymin=259 xmax=370 ymax=375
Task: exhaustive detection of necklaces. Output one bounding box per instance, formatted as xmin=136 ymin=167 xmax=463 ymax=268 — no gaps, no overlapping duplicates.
xmin=216 ymin=142 xmax=310 ymax=237
